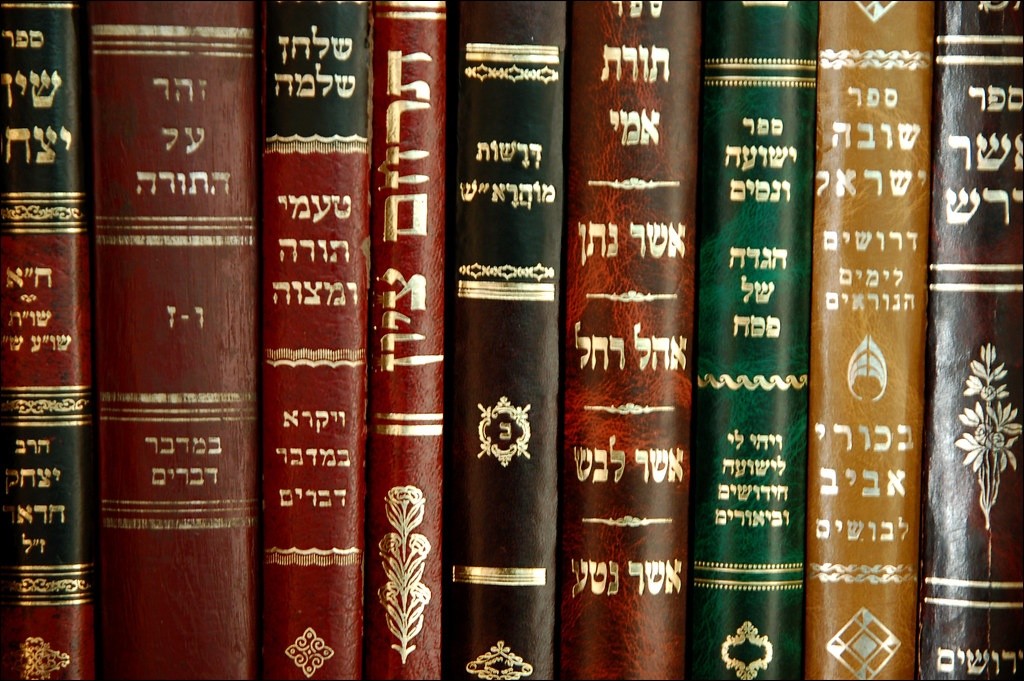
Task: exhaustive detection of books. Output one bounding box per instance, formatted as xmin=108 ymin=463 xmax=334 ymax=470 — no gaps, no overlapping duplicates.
xmin=0 ymin=0 xmax=1024 ymax=681
